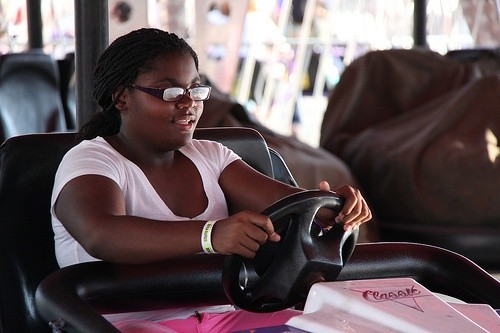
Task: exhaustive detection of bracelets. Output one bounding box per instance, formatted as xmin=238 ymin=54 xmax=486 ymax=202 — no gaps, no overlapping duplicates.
xmin=201 ymin=220 xmax=218 ymax=255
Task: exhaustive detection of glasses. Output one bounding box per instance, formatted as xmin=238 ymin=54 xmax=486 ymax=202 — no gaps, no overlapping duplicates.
xmin=125 ymin=83 xmax=211 ymax=103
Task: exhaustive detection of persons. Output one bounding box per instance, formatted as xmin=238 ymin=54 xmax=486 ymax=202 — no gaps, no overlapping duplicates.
xmin=1 ymin=1 xmax=473 ymax=150
xmin=49 ymin=27 xmax=373 ymax=332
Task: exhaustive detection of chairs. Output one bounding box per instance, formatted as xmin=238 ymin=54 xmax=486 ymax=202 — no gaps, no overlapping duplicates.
xmin=0 ymin=52 xmax=68 ymax=137
xmin=0 ymin=127 xmax=302 ymax=332
